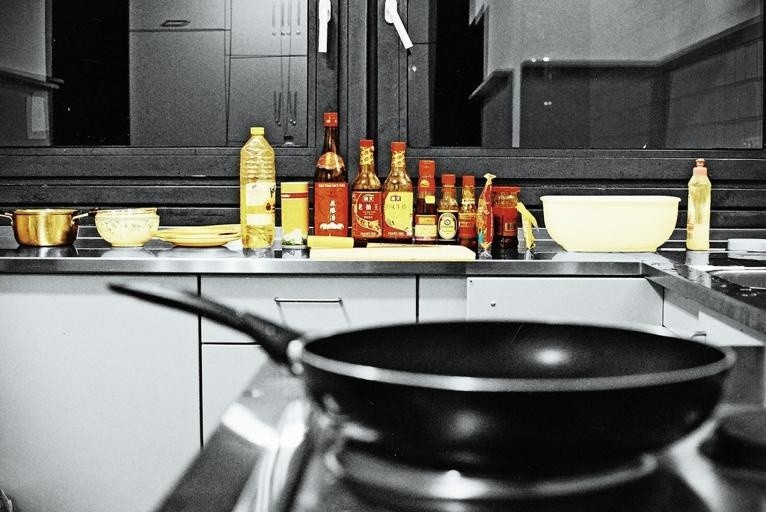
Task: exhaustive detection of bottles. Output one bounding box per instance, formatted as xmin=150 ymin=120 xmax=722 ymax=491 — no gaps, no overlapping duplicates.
xmin=237 ymin=111 xmax=478 ymax=249
xmin=685 ymin=157 xmax=712 ymax=251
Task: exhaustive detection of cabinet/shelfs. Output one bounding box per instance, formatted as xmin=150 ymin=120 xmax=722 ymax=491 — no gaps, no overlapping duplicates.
xmin=127 ymin=1 xmax=308 ymax=146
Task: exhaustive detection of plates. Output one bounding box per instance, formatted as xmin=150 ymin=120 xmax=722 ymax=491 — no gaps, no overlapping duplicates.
xmin=148 ymin=224 xmax=246 ymax=252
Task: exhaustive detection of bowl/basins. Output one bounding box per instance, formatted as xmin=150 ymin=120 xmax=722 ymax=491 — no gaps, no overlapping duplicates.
xmin=89 ymin=208 xmax=159 ymax=249
xmin=537 ymin=192 xmax=683 ymax=253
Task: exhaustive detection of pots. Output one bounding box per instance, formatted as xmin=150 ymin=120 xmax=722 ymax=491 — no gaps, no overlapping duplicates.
xmin=2 ymin=206 xmax=99 ymax=251
xmin=99 ymin=273 xmax=734 ymax=487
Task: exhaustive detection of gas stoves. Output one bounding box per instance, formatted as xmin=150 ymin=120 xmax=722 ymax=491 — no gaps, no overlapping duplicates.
xmin=143 ymin=345 xmax=766 ymax=512
